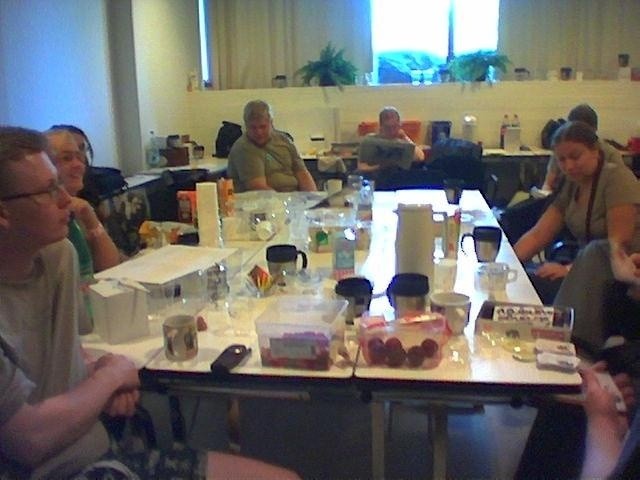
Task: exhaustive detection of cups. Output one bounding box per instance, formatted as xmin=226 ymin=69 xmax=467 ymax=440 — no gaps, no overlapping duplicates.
xmin=477 ymin=262 xmax=519 ymax=292
xmin=384 ymin=271 xmax=430 ymax=324
xmin=161 ymin=314 xmax=201 ymax=363
xmin=333 ymin=275 xmax=375 ymax=327
xmin=442 ymin=184 xmax=465 ymax=206
xmin=263 ymin=242 xmax=310 ymax=289
xmin=430 ymin=289 xmax=472 ymax=337
xmin=322 ymin=177 xmax=344 ymax=197
xmin=460 ymin=224 xmax=504 ymax=263
xmin=253 ymin=217 xmax=275 ymax=241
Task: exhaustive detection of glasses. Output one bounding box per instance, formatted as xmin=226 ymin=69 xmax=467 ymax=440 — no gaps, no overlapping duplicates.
xmin=1 ymin=181 xmax=57 ymax=206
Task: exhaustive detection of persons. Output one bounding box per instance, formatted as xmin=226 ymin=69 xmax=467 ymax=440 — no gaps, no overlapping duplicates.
xmin=225 ymin=99 xmax=320 ymax=195
xmin=40 ymin=128 xmax=126 ymax=336
xmin=510 ymin=101 xmax=640 ymax=480
xmin=51 ymin=123 xmax=109 ymax=223
xmin=355 ymin=106 xmax=426 ymax=191
xmin=1 ymin=124 xmax=303 ymax=480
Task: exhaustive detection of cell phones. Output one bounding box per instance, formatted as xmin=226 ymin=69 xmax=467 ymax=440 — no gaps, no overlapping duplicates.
xmin=210 ymin=344 xmax=252 ymax=374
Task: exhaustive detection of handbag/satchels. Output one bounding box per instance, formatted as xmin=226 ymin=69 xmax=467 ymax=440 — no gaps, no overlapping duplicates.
xmin=212 ymin=121 xmax=242 ymax=158
xmin=89 ymin=163 xmax=126 ymax=195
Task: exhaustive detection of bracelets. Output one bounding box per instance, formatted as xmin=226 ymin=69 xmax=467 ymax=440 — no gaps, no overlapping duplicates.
xmin=82 ymin=221 xmax=104 ymax=240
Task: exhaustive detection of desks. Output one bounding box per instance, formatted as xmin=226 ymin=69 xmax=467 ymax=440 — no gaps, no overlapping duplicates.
xmin=144 ymin=156 xmax=229 ymax=183
xmin=79 ymin=188 xmax=585 ymax=480
xmin=481 ymin=145 xmax=553 ymax=174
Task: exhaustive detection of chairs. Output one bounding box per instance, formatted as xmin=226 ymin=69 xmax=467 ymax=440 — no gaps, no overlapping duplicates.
xmin=421 ymin=138 xmax=499 ymax=208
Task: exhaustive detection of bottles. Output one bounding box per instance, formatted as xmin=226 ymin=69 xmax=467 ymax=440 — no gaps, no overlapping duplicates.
xmin=498 ymin=112 xmax=521 ymax=148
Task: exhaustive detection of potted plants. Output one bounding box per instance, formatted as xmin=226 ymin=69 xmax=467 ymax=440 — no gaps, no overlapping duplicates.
xmin=447 ymin=48 xmax=516 ymax=90
xmin=291 ymin=39 xmax=360 ymax=94
